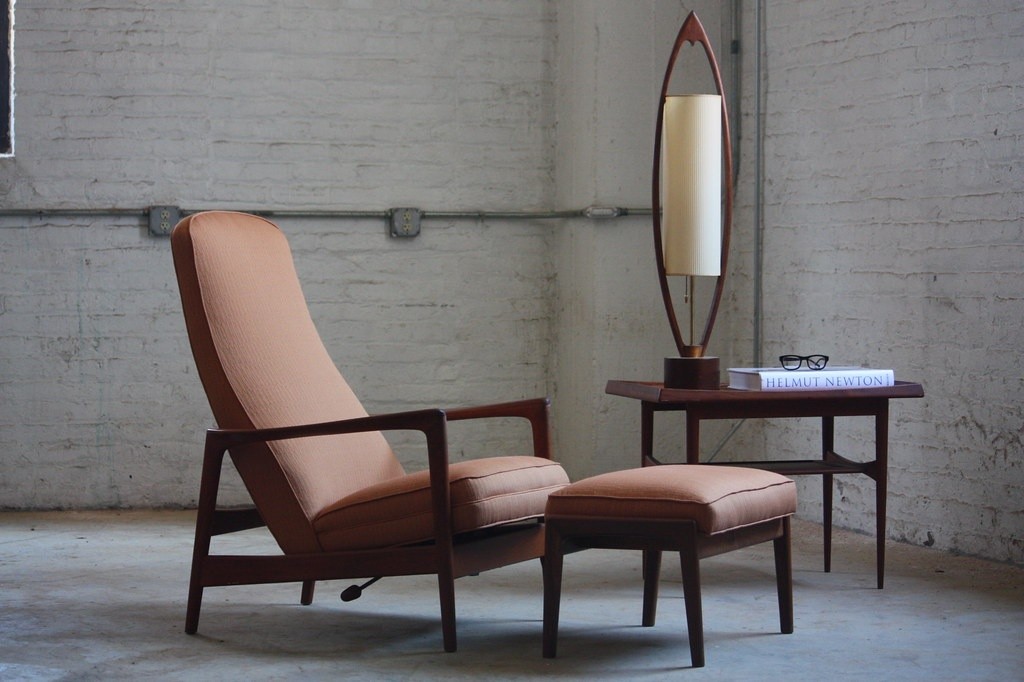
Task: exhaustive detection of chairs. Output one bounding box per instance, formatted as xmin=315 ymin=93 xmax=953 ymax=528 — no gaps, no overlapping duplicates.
xmin=170 ymin=209 xmax=573 ymax=654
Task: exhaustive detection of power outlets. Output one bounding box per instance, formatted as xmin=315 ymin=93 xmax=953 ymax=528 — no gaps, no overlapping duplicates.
xmin=147 ymin=205 xmax=184 ymax=239
xmin=389 ymin=208 xmax=422 ymax=237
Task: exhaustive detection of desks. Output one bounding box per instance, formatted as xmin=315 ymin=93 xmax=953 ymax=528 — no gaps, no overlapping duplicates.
xmin=605 ymin=379 xmax=926 ymax=591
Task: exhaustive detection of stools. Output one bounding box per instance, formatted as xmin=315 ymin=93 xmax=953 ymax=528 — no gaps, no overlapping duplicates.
xmin=546 ymin=465 xmax=797 ymax=667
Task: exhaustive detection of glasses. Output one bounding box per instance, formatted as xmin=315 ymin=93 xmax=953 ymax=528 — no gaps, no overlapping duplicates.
xmin=779 ymin=354 xmax=829 ymax=371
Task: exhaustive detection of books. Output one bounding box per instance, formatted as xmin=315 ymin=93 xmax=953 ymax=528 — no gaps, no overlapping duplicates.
xmin=727 ymin=366 xmax=895 ymax=391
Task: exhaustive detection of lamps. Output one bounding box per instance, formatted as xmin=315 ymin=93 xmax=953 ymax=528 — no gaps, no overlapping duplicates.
xmin=652 ymin=9 xmax=734 ymax=390
